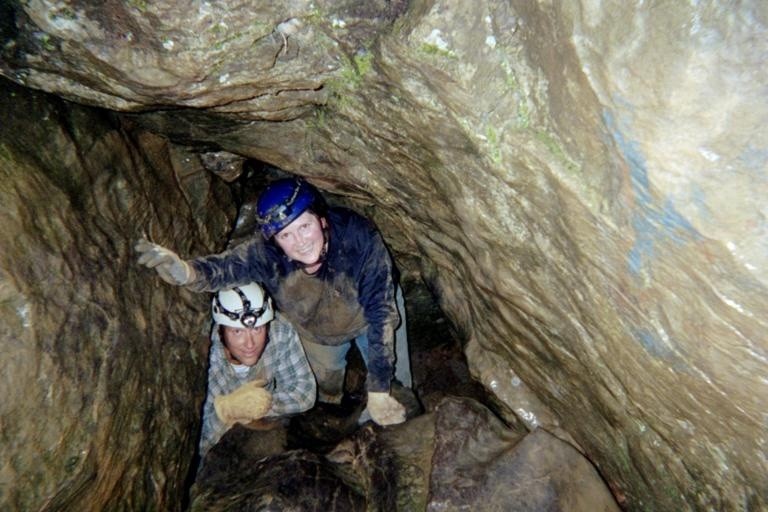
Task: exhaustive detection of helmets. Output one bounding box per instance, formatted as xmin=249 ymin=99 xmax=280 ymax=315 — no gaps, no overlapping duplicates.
xmin=253 ymin=178 xmax=319 ymax=244
xmin=211 ymin=275 xmax=276 ymax=330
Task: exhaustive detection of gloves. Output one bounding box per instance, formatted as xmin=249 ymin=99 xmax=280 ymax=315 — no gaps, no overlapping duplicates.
xmin=357 ymin=391 xmax=408 ymax=427
xmin=134 ymin=236 xmax=192 ymax=289
xmin=213 ymin=378 xmax=273 ymax=426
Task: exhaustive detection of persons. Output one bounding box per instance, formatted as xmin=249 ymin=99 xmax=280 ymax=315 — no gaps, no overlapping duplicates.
xmin=188 ymin=279 xmax=318 ymax=478
xmin=134 ymin=174 xmax=412 ymax=425
